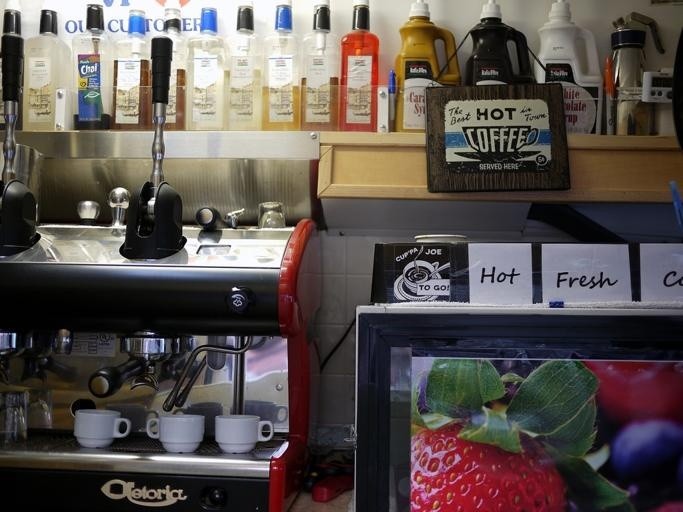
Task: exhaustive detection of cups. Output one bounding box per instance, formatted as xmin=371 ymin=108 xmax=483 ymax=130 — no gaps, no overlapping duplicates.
xmin=214 ymin=414 xmax=274 ymax=454
xmin=74 ymin=409 xmax=131 ymax=448
xmin=146 ymin=414 xmax=204 ymax=453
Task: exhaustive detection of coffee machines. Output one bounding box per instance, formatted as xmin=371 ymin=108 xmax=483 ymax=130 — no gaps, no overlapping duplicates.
xmin=1 ymin=36 xmax=324 ymax=512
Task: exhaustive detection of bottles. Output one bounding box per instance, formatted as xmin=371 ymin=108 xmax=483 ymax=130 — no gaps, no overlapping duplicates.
xmin=301 ymin=1 xmax=337 ymax=131
xmin=23 ymin=11 xmax=72 ymax=132
xmin=262 ymin=1 xmax=300 ymax=131
xmin=339 ymin=1 xmax=380 ymax=134
xmin=148 ymin=8 xmax=187 ymax=132
xmin=111 ymin=9 xmax=150 ymax=129
xmin=72 ymin=5 xmax=113 ymax=131
xmin=1 ymin=9 xmax=23 ymax=130
xmin=226 ymin=6 xmax=262 ymax=131
xmin=186 ymin=8 xmax=226 ymax=130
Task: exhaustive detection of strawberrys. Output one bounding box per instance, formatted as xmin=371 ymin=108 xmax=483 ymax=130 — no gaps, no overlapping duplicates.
xmin=411 ymin=358 xmax=630 ymax=512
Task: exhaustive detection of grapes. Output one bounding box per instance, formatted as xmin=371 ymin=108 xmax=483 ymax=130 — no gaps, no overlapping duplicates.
xmin=614 ymin=418 xmax=683 ymax=480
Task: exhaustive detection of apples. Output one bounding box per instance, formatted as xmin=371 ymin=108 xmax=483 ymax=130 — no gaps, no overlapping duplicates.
xmin=584 ymin=361 xmax=683 ymax=419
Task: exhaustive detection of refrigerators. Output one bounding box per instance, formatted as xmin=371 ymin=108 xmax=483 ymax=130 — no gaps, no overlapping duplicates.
xmin=353 ymin=301 xmax=683 ymax=512
